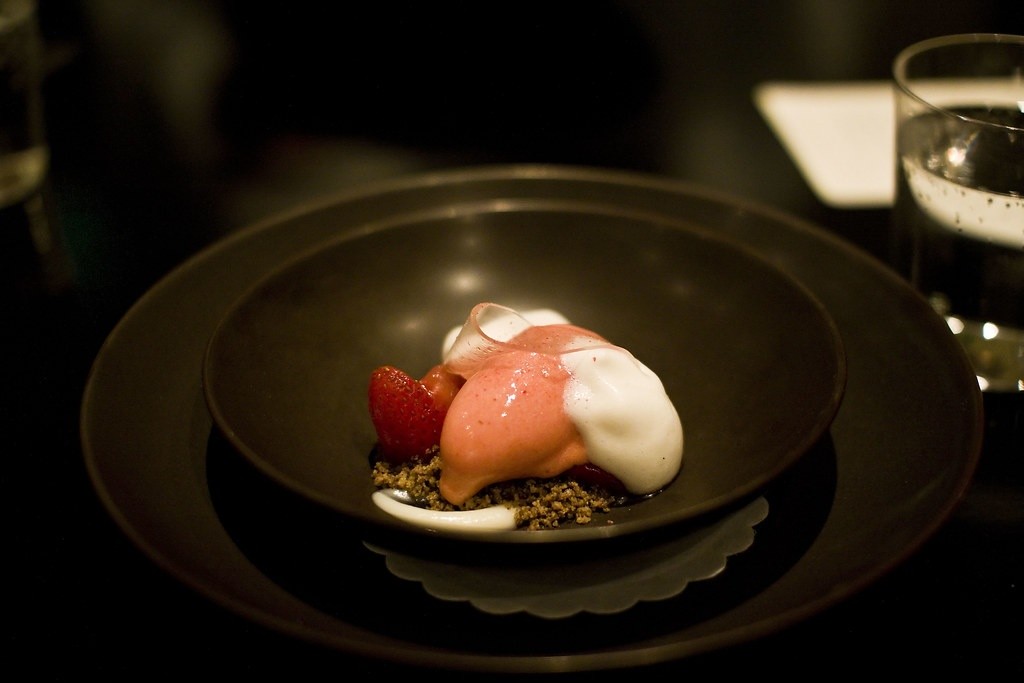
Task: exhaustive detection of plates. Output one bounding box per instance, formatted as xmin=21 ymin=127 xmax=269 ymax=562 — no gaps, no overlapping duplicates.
xmin=81 ymin=163 xmax=984 ymax=675
xmin=201 ymin=200 xmax=848 ymax=546
xmin=752 ymin=77 xmax=1024 ymax=208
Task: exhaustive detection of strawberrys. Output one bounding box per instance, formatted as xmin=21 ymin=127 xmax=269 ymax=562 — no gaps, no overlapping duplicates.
xmin=365 ymin=364 xmax=447 ymax=463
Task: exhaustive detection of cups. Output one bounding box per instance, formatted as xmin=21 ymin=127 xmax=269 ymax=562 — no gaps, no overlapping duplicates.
xmin=0 ymin=0 xmax=49 ymax=207
xmin=889 ymin=33 xmax=1024 ymax=406
